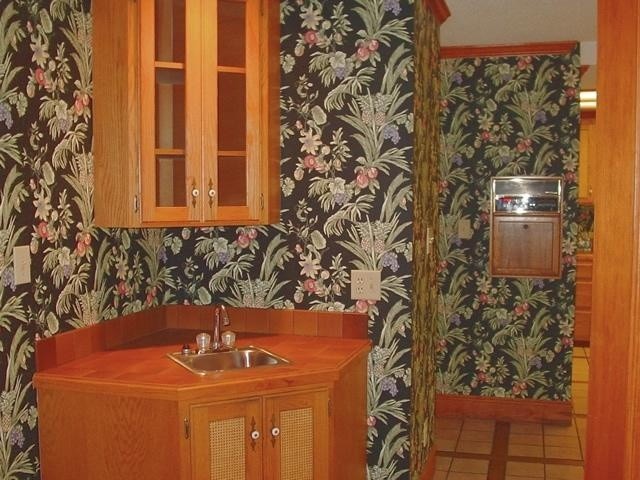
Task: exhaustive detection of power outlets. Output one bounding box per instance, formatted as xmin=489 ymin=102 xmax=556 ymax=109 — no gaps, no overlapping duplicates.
xmin=351 ymin=270 xmax=382 ymax=301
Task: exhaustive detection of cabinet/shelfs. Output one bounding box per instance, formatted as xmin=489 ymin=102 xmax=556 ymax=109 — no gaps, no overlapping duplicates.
xmin=579 ymin=119 xmax=596 ymax=205
xmin=492 ymin=212 xmax=562 ymax=279
xmin=36 ymin=357 xmax=367 ymax=480
xmin=576 ymin=260 xmax=593 ymax=342
xmin=91 ymin=0 xmax=280 ymax=229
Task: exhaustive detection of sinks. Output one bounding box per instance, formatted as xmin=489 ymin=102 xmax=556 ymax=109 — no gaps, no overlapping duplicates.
xmin=168 ymin=345 xmax=293 ymax=376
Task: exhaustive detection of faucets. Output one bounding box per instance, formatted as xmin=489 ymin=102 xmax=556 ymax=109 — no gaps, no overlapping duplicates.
xmin=213 ymin=304 xmax=229 ymax=346
